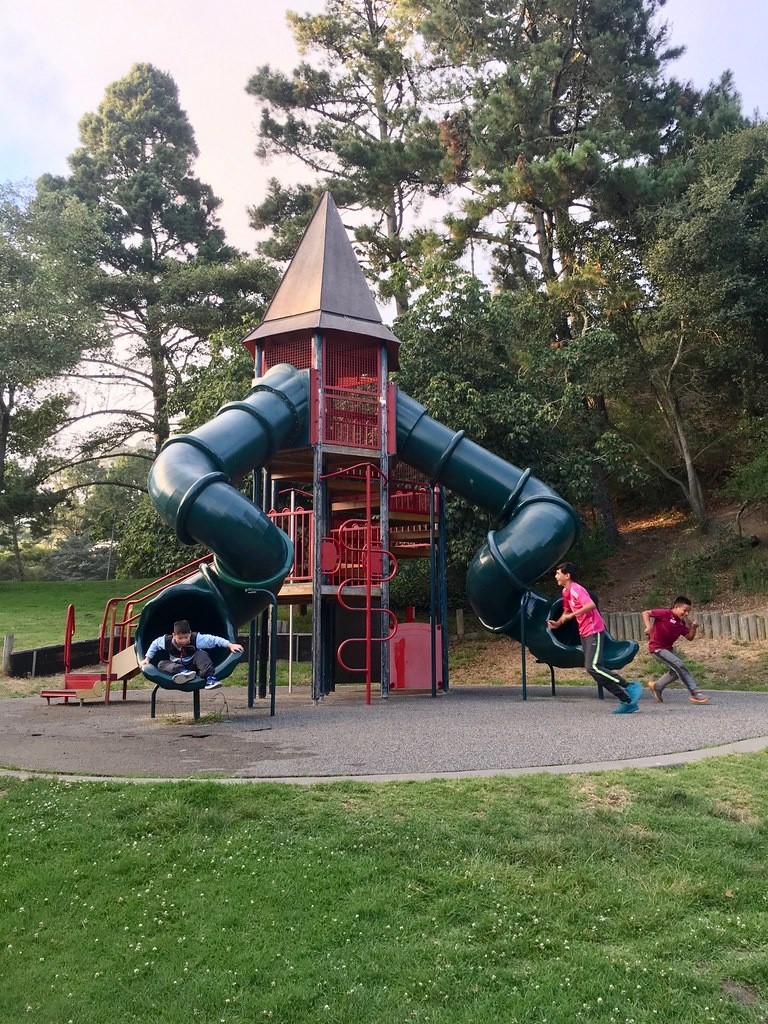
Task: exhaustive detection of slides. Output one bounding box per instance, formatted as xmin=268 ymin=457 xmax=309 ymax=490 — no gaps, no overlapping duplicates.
xmin=396 ymin=389 xmax=641 ymax=670
xmin=132 ymin=361 xmax=314 ymax=692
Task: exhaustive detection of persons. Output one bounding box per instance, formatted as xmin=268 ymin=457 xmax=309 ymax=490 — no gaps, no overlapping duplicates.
xmin=141 ymin=620 xmax=244 ymax=690
xmin=643 ymin=596 xmax=711 ymax=703
xmin=547 ymin=562 xmax=642 ymax=714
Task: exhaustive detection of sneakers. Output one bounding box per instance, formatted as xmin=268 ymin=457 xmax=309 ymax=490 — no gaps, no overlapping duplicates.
xmin=625 ymin=680 xmax=644 ymax=704
xmin=689 ymin=692 xmax=709 ymax=702
xmin=172 ymin=669 xmax=196 ymax=683
xmin=204 ymin=676 xmax=223 ymax=690
xmin=647 ymin=681 xmax=664 ymax=702
xmin=611 ymin=697 xmax=640 ymax=713
